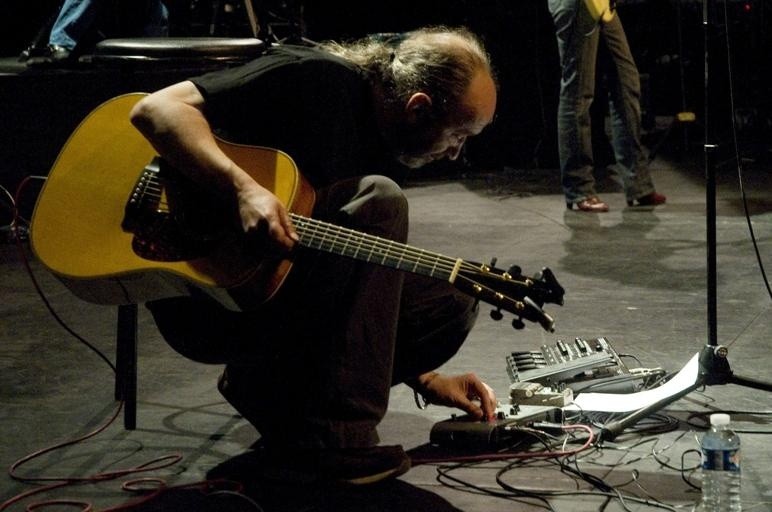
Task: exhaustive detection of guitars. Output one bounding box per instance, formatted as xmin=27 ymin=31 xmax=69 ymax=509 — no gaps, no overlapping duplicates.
xmin=27 ymin=90 xmax=565 ymax=334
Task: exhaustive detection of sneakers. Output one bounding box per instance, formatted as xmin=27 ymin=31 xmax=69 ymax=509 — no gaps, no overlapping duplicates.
xmin=219 ymin=365 xmax=290 ymax=440
xmin=271 ymin=436 xmax=410 ymax=484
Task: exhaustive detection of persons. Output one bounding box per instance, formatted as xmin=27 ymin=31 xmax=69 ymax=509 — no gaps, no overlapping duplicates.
xmin=547 ymin=0 xmax=666 ymax=212
xmin=128 ymin=26 xmax=497 ymax=485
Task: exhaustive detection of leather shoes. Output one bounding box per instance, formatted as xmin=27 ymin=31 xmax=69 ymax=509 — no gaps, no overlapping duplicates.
xmin=627 ymin=189 xmax=664 ymax=207
xmin=568 ymin=193 xmax=609 ymax=213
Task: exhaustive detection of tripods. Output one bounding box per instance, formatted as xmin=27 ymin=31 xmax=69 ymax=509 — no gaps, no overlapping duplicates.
xmin=603 ymin=0 xmax=772 ymax=442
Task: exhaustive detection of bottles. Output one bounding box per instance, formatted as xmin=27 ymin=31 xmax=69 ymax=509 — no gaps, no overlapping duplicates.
xmin=701 ymin=413 xmax=742 ymax=512
